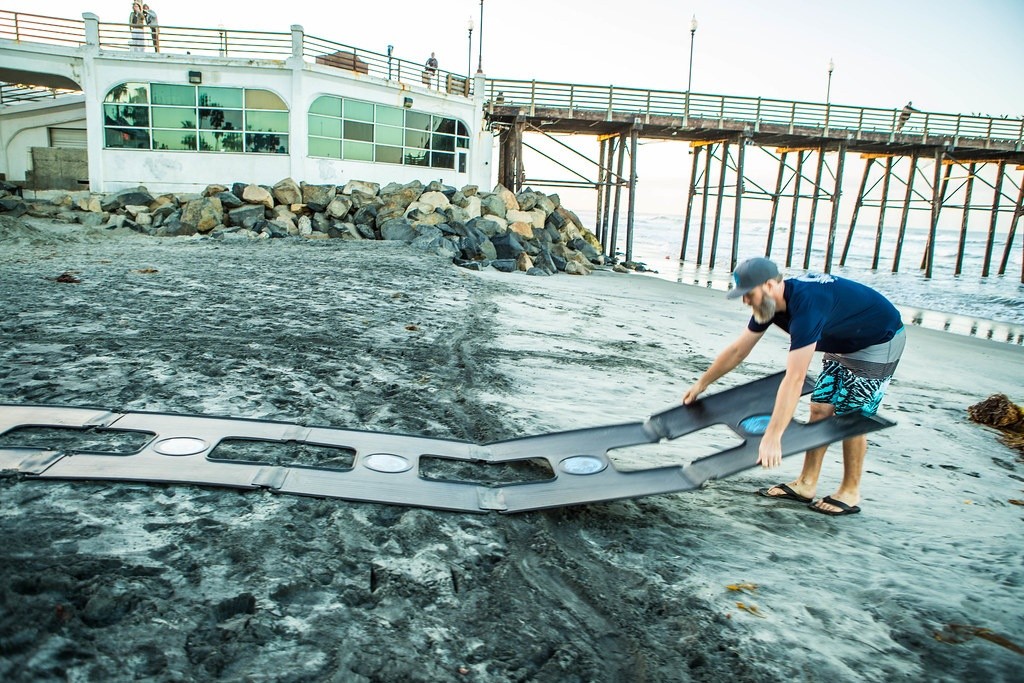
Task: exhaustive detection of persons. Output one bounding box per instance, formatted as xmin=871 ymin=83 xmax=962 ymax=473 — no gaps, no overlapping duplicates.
xmin=142 ymin=4 xmax=159 ymax=53
xmin=128 ymin=2 xmax=145 ymax=52
xmin=683 ymin=258 xmax=907 ymax=515
xmin=896 ymin=101 xmax=919 ymax=131
xmin=424 ymin=52 xmax=437 ymax=89
xmin=496 ymin=91 xmax=504 ymax=104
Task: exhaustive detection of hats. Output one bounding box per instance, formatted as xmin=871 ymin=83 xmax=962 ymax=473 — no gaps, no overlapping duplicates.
xmin=727 ymin=257 xmax=779 ymax=298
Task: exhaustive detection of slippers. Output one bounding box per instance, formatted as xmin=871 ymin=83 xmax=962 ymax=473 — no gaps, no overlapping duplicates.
xmin=810 ymin=496 xmax=860 ymax=515
xmin=760 ymin=484 xmax=812 ymax=503
